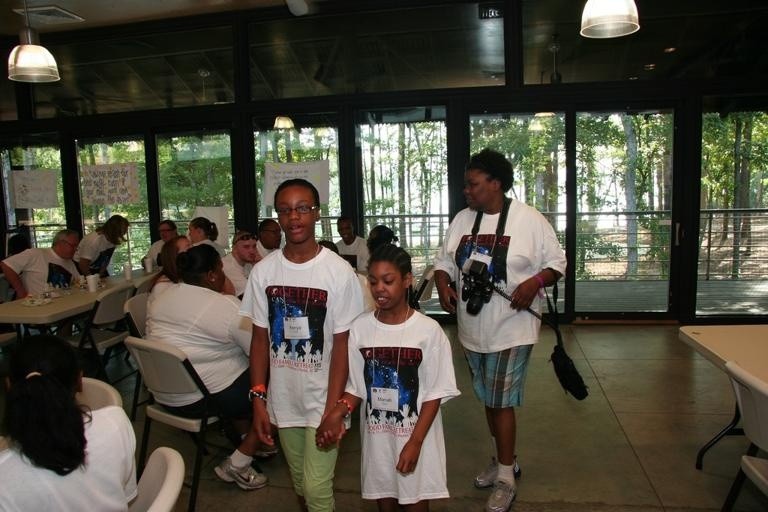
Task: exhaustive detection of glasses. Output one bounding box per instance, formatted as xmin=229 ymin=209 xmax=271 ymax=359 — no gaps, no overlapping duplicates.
xmin=276 ymin=204 xmax=318 ymax=214
xmin=230 ymin=234 xmax=260 ymax=243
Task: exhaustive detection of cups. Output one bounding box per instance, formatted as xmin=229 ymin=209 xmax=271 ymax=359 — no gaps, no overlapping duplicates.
xmin=143 ymin=259 xmax=152 ymax=273
xmin=124 ymin=265 xmax=132 ymax=281
xmin=86 ymin=276 xmax=96 ymax=292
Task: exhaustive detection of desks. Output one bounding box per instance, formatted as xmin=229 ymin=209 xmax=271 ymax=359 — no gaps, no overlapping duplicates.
xmin=678 ymin=323 xmax=768 ymax=470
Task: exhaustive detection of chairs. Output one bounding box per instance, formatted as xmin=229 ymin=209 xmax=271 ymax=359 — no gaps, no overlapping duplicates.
xmin=121 ymin=334 xmax=248 ymax=512
xmin=134 ymin=272 xmax=161 ymax=295
xmin=122 ymin=293 xmax=150 ymax=422
xmin=411 ymin=263 xmax=436 ymax=316
xmin=718 ymin=359 xmax=768 ymax=512
xmin=65 ymin=280 xmax=136 ymax=392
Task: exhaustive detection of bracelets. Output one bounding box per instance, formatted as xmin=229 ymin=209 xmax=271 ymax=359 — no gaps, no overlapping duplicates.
xmin=248 ymin=385 xmax=267 ymax=400
xmin=335 ymin=400 xmax=352 ymax=417
xmin=534 ymin=275 xmax=544 ymax=287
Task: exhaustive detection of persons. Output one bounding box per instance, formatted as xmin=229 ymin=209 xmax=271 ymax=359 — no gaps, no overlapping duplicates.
xmin=2 ymin=335 xmax=138 ymax=510
xmin=433 ymin=150 xmax=567 ymax=511
xmin=315 ymin=244 xmax=461 ymax=510
xmin=239 ymin=179 xmax=364 ymax=511
xmin=145 ymin=243 xmax=267 ymax=490
xmin=0 ymin=215 xmax=397 ymax=300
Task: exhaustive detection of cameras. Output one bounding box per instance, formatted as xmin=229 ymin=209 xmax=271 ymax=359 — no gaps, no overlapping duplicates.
xmin=460 ymin=258 xmax=495 ymax=316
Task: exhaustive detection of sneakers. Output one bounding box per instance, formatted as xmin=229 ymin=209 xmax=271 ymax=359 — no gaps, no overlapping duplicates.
xmin=239 ymin=435 xmax=279 ymax=458
xmin=484 ymin=478 xmax=517 ymax=512
xmin=471 ymin=457 xmax=522 ymax=488
xmin=211 ymin=455 xmax=269 ymax=491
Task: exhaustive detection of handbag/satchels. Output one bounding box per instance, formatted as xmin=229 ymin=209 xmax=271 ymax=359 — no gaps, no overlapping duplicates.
xmin=550 ymin=343 xmax=590 ymax=400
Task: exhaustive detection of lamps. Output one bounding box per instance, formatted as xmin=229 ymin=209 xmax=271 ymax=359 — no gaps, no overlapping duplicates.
xmin=6 ymin=0 xmax=64 ymax=85
xmin=580 ymin=0 xmax=640 ymax=41
xmin=273 ymin=114 xmax=295 ymax=131
